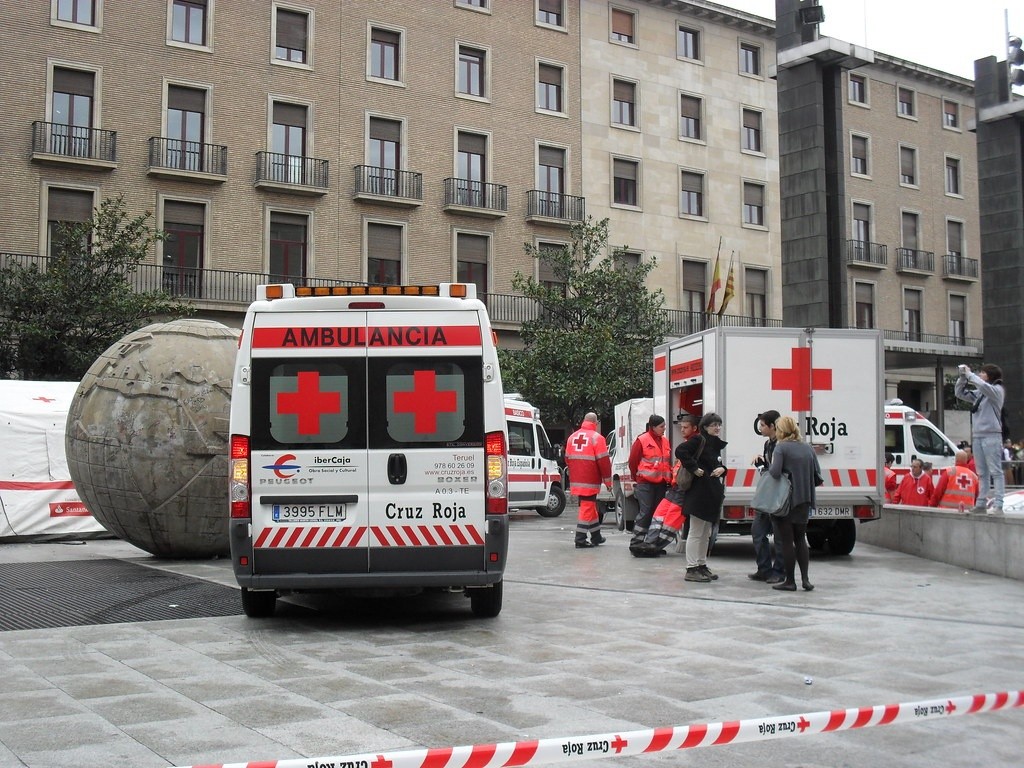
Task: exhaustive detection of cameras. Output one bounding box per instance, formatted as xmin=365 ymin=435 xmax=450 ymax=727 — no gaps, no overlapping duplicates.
xmin=958 ymin=364 xmax=968 ymax=374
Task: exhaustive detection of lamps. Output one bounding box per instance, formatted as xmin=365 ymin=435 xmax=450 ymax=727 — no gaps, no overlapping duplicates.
xmin=1008 ymin=36 xmax=1024 ymax=86
xmin=800 ymin=4 xmax=824 ymax=26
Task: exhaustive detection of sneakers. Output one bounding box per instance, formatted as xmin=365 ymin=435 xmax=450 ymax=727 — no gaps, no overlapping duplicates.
xmin=697 ymin=565 xmax=718 ymax=580
xmin=685 ymin=568 xmax=711 ymax=582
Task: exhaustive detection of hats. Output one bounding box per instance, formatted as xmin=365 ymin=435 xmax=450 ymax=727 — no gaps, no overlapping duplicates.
xmin=650 ymin=415 xmax=664 ymax=427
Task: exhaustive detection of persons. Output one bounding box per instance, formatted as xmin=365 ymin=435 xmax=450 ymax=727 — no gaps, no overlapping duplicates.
xmin=767 ymin=416 xmax=821 ymax=591
xmin=675 ymin=413 xmax=728 ymax=582
xmin=954 ymin=365 xmax=1005 ymax=516
xmin=629 ymin=415 xmax=670 ymax=554
xmin=747 ymin=410 xmax=789 ymax=584
xmin=884 ymin=438 xmax=1024 ymax=512
xmin=565 ymin=413 xmax=612 ymax=548
xmin=629 ymin=416 xmax=705 ymax=557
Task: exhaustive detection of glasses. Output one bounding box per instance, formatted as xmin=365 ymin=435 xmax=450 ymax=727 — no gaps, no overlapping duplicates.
xmin=708 ymin=423 xmax=722 ymax=428
xmin=910 ymin=465 xmax=922 ymax=469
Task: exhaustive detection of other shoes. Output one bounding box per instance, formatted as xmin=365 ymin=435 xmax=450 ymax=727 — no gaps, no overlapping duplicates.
xmin=767 ymin=576 xmax=784 ymax=583
xmin=592 ymin=537 xmax=606 ymax=545
xmin=748 ymin=571 xmax=768 ymax=581
xmin=989 ymin=508 xmax=1003 ymax=517
xmin=576 ymin=542 xmax=594 ymax=548
xmin=773 ymin=581 xmax=796 ymax=591
xmin=803 ymin=584 xmax=815 ymax=591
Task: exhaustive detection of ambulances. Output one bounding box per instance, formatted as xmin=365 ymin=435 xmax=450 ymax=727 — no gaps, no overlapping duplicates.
xmin=596 ymin=326 xmax=886 ymax=558
xmin=884 ymin=398 xmax=965 ymax=489
xmin=227 ymin=283 xmax=509 ymax=621
xmin=502 ymin=392 xmax=567 ymax=519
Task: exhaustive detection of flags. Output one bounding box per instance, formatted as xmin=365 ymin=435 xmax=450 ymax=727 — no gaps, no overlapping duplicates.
xmin=707 ymin=246 xmax=722 ymax=320
xmin=717 ymin=253 xmax=736 ymax=323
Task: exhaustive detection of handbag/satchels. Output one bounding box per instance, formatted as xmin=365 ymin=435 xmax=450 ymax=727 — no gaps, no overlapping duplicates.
xmin=675 ymin=435 xmax=706 ymax=490
xmin=749 ymin=466 xmax=791 ymax=517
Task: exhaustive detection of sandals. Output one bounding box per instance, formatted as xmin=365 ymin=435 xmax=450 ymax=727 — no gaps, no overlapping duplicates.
xmin=965 ymin=508 xmax=987 ymax=516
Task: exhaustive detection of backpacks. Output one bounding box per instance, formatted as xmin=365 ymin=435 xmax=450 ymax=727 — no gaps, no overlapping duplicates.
xmin=991 ymin=405 xmax=1010 ymax=441
